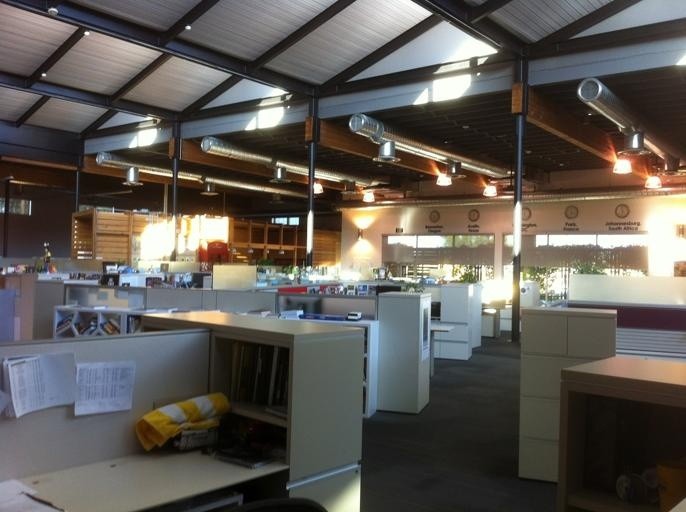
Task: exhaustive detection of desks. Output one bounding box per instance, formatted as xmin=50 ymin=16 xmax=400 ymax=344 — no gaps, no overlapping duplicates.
xmin=431 ymin=327 xmax=455 ymax=379
xmin=0 ymin=449 xmax=290 ymax=512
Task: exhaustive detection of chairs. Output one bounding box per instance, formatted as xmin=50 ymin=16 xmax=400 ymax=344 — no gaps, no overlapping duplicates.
xmin=226 ymin=497 xmax=328 ymax=512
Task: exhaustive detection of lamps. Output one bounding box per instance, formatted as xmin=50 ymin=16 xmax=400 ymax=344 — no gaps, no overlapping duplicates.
xmin=436 ymin=171 xmax=452 ymax=187
xmin=233 ymin=248 xmax=236 ymax=253
xmin=267 ymin=250 xmax=270 ymax=254
xmin=612 ymin=158 xmax=632 ymax=174
xmin=358 ymin=228 xmax=363 ymax=240
xmin=362 ymin=191 xmax=375 ymax=203
xmin=314 ymin=183 xmax=323 ymax=194
xmin=248 ymin=248 xmax=254 ymax=254
xmin=278 ymin=250 xmax=285 ymax=254
xmin=644 ymin=176 xmax=662 ymax=190
xmin=482 ymin=184 xmax=497 ymax=197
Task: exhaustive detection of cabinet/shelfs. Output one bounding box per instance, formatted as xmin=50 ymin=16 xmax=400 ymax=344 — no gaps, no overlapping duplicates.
xmin=124 ymin=311 xmax=220 ymax=335
xmin=519 ymin=309 xmax=618 ymax=482
xmin=556 ymin=356 xmax=686 ymax=512
xmin=5 ymin=273 xmax=38 ymax=340
xmin=299 ymin=319 xmax=380 ymax=418
xmin=377 ymin=291 xmax=432 ymax=414
xmin=53 ymin=304 xmax=124 ymax=339
xmin=140 ymin=312 xmax=365 ymax=512
xmin=20 ymin=279 xmax=64 ymax=340
xmin=433 ymin=283 xmax=472 ymax=360
xmin=500 ymin=281 xmax=540 ymax=331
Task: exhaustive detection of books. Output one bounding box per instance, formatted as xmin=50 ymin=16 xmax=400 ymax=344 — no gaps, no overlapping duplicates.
xmin=213 ymin=341 xmax=289 ymax=469
xmin=54 ymin=313 xmax=143 ymax=335
xmin=298 ymin=312 xmax=347 ymax=322
